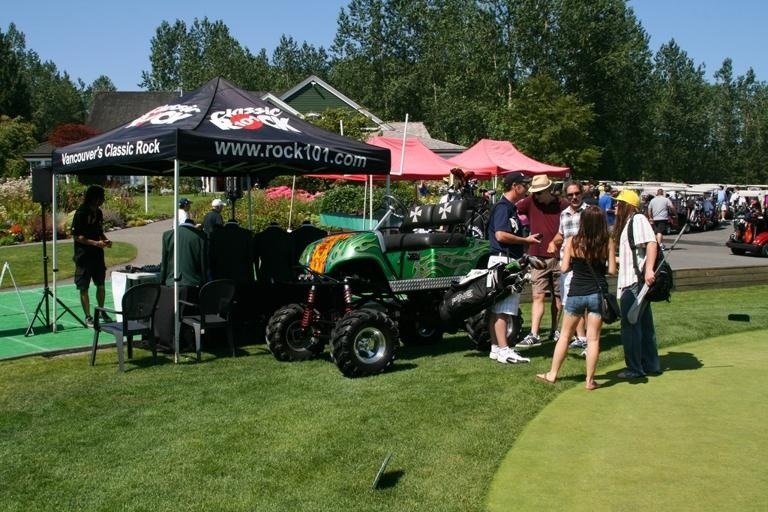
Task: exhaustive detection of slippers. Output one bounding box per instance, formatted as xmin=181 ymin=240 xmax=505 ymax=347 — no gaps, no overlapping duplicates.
xmin=537 ymin=372 xmax=556 ymax=384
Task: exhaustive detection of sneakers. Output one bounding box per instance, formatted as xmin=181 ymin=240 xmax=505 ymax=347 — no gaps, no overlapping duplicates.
xmin=618 ymin=369 xmax=641 ymax=379
xmin=86 ymin=316 xmax=94 ymax=327
xmin=100 ymin=311 xmax=112 ymax=323
xmin=549 ymin=331 xmax=589 ymax=357
xmin=514 ymin=332 xmax=543 ymax=348
xmin=489 ymin=344 xmax=531 ymax=363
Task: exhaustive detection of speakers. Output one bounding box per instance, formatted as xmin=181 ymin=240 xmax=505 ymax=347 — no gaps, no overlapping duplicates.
xmin=226 ymin=176 xmax=242 ymax=200
xmin=32 ymin=167 xmax=52 ymax=204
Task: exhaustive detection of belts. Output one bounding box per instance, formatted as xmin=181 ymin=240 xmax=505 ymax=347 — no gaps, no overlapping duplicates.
xmin=492 ymin=251 xmax=508 ymax=256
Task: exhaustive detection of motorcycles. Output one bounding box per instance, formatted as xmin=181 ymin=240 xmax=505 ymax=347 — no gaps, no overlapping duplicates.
xmin=725 ymin=207 xmax=768 ymax=258
xmin=642 ymin=195 xmax=721 ymax=233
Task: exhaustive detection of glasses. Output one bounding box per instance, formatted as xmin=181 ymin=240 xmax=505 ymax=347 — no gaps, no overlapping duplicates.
xmin=567 ymin=191 xmax=581 ymax=199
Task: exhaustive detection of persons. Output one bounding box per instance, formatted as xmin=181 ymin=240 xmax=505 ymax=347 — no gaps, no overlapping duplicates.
xmin=68 ymin=183 xmax=113 ymax=329
xmin=611 ymin=188 xmax=665 ymax=381
xmin=201 ymin=197 xmax=229 ymax=239
xmin=415 ymin=171 xmax=767 ymax=365
xmin=534 ymin=204 xmax=618 ymax=391
xmin=176 ymin=196 xmax=193 ymax=226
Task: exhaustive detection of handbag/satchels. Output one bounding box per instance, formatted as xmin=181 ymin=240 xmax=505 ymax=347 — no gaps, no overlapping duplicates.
xmin=600 ymin=293 xmax=620 ymax=324
xmin=74 ymin=247 xmax=97 ymax=269
xmin=634 ymin=245 xmax=673 ymax=301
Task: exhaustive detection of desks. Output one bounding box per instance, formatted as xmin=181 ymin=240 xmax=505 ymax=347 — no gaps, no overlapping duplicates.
xmin=110 ymin=269 xmax=162 ymax=321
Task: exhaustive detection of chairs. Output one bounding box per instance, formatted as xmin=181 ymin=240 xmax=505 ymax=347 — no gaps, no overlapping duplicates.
xmin=88 ymin=283 xmax=163 ymax=373
xmin=179 ymin=278 xmax=239 ymax=363
xmin=383 ymin=204 xmax=436 ymax=249
xmin=402 ymin=199 xmax=466 ymax=248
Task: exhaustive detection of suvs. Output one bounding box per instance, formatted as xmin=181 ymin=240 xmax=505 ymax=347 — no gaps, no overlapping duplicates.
xmin=267 ymin=195 xmax=524 ymax=378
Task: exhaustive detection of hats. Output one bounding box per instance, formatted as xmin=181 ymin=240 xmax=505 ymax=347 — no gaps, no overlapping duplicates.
xmin=504 ymin=172 xmax=532 ymax=184
xmin=179 ymin=198 xmax=193 ymax=205
xmin=528 ymin=174 xmax=552 ymax=192
xmin=212 ymin=199 xmax=227 ymax=206
xmin=612 ymin=190 xmax=640 ymax=208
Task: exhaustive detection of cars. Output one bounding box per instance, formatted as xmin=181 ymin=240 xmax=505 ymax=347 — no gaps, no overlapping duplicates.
xmin=552 ymin=181 xmax=768 ymax=198
xmin=729 ymin=188 xmax=768 ymax=214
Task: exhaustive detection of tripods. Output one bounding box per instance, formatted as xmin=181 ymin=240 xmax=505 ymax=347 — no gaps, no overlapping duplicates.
xmin=24 ymin=259 xmax=88 ymax=337
